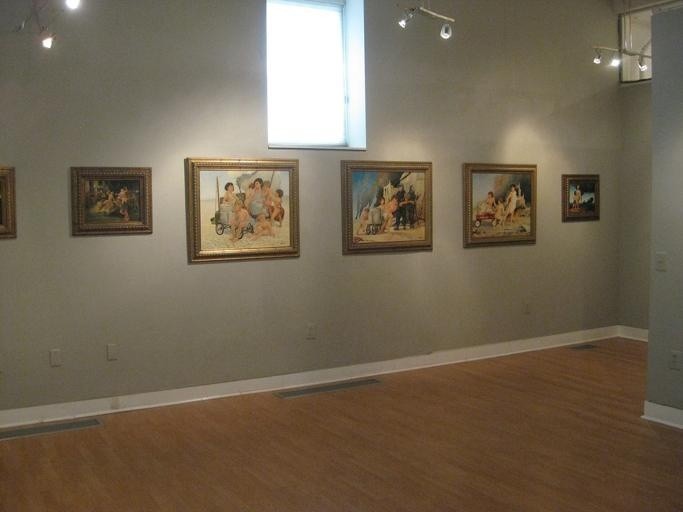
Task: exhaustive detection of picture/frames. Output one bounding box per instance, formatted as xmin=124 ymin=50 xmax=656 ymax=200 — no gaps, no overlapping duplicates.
xmin=0 ymin=165 xmax=18 ymax=241
xmin=560 ymin=173 xmax=602 ymax=223
xmin=461 ymin=162 xmax=538 ymax=249
xmin=183 ymin=156 xmax=301 ymax=266
xmin=339 ymin=159 xmax=434 ymax=257
xmin=68 ymin=166 xmax=154 ymax=238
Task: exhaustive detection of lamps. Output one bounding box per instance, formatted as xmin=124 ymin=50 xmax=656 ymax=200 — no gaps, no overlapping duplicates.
xmin=591 ymin=40 xmax=651 ymax=73
xmin=397 ymin=1 xmax=457 ymax=41
